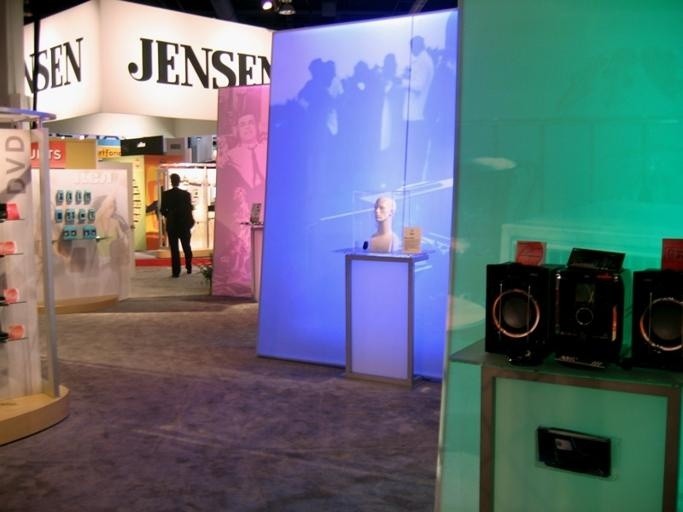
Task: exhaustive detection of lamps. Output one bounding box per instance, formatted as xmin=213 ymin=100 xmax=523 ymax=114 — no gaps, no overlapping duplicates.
xmin=260 ymin=0 xmax=296 ymax=15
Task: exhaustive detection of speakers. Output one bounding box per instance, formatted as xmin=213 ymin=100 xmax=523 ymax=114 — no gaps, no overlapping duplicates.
xmin=485 ymin=262 xmax=566 ymax=367
xmin=631 ymin=270 xmax=683 ymax=373
xmin=555 ymin=268 xmax=634 ymax=371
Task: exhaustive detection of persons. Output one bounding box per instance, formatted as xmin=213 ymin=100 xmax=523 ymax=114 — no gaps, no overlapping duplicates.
xmin=99 ymin=236 xmax=130 ymax=293
xmin=266 ymin=8 xmax=460 ymax=213
xmin=224 ymin=110 xmax=266 ymax=197
xmin=91 ymin=194 xmax=132 ymax=292
xmin=158 ymin=171 xmax=196 ymax=279
xmin=365 ymin=195 xmax=403 ymax=252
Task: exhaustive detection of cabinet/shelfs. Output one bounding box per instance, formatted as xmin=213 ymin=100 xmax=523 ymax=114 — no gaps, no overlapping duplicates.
xmin=0 ymin=217 xmax=29 ymax=342
